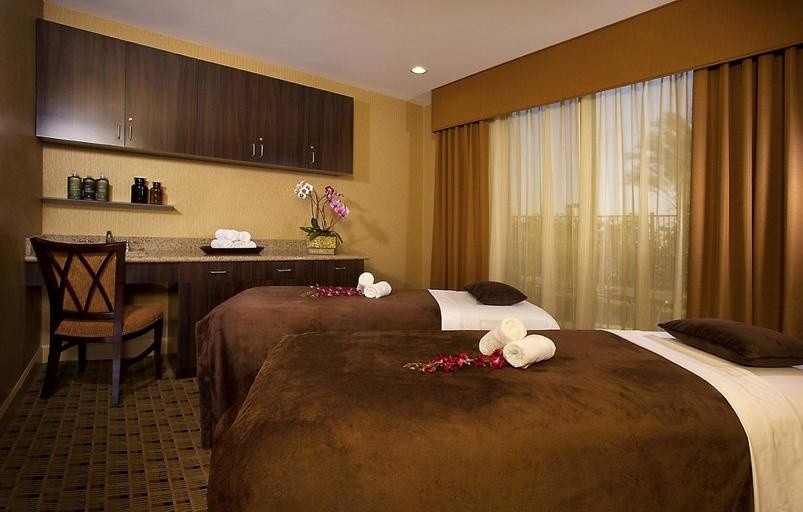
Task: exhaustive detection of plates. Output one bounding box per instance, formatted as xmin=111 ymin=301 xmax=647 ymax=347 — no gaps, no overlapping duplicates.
xmin=200 ymin=246 xmax=264 ymax=256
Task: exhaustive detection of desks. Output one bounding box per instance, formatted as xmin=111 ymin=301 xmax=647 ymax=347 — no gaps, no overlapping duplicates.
xmin=24 ymin=234 xmax=372 ymax=380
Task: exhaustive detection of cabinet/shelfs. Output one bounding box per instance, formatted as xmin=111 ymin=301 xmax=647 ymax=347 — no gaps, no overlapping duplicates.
xmin=198 ymin=56 xmax=292 ymax=177
xmin=35 ymin=17 xmax=200 ymax=156
xmin=292 ymin=81 xmax=353 ymax=176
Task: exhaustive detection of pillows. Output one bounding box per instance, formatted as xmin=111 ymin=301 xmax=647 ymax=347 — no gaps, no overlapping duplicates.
xmin=463 ymin=281 xmax=528 ymax=306
xmin=657 ymin=318 xmax=803 ymax=368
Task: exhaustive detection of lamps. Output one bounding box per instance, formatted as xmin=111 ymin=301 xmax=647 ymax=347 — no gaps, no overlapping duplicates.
xmin=410 ymin=66 xmax=428 ymax=74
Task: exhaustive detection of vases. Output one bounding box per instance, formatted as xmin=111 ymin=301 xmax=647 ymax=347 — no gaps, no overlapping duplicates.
xmin=306 ymin=235 xmax=337 ymax=255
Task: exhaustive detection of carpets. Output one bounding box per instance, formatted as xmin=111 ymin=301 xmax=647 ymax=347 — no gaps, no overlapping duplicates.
xmin=1 ymin=352 xmax=211 ymax=512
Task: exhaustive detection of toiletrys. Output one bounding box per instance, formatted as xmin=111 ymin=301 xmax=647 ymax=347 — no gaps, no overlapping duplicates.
xmin=82 ymin=173 xmax=95 ymax=200
xmin=67 ymin=168 xmax=82 ymax=200
xmin=96 ymin=173 xmax=109 ymax=201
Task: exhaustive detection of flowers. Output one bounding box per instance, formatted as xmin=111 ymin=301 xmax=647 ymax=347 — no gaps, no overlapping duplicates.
xmin=403 ymin=348 xmax=505 ymax=373
xmin=293 ymin=181 xmax=351 ymax=243
xmin=302 ymin=284 xmax=362 ymax=298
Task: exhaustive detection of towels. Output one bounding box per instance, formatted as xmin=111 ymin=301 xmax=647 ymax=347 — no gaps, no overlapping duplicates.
xmin=247 ymin=241 xmax=255 ymax=248
xmin=364 ymin=281 xmax=391 ymax=298
xmin=211 ymin=240 xmax=234 ymax=249
xmin=503 ymin=334 xmax=556 ymax=368
xmin=357 ymin=272 xmax=374 ymax=290
xmin=215 ymin=229 xmax=239 ymax=241
xmin=479 ymin=317 xmax=526 ymax=355
xmin=239 ymin=231 xmax=250 ymax=241
xmin=233 ymin=242 xmax=245 ymax=248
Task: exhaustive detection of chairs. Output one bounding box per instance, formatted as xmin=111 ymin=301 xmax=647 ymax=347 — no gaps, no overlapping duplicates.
xmin=29 ymin=236 xmax=164 ymax=408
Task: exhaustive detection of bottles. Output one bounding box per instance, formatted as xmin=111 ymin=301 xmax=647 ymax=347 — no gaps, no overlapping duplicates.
xmin=82 ymin=176 xmax=95 ymax=200
xmin=131 ymin=178 xmax=148 ymax=203
xmin=95 ymin=176 xmax=108 ymax=201
xmin=68 ymin=170 xmax=81 ymax=199
xmin=150 ymin=182 xmax=162 ymax=205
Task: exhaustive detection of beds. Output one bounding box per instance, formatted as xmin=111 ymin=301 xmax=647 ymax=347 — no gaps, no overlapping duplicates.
xmin=207 ymin=328 xmax=803 ymax=512
xmin=194 ymin=286 xmax=560 ymax=458
